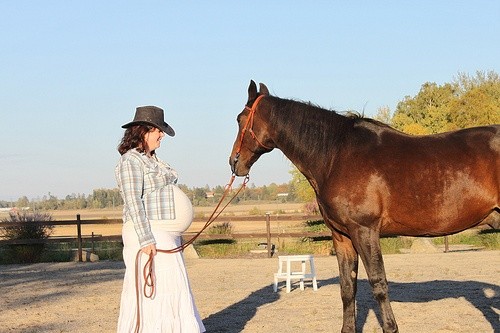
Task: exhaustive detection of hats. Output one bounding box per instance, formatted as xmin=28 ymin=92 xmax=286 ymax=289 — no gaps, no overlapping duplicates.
xmin=121 ymin=105 xmax=174 ymax=136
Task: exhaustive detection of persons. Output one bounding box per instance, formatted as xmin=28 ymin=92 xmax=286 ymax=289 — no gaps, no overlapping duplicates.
xmin=115 ymin=106 xmax=206 ymax=333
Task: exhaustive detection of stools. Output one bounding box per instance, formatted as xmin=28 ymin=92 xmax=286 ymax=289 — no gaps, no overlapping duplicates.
xmin=274 ymin=255 xmax=318 ymax=293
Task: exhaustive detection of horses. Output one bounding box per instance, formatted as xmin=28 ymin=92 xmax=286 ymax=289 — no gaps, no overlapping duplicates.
xmin=226 ymin=76 xmax=500 ymax=332
